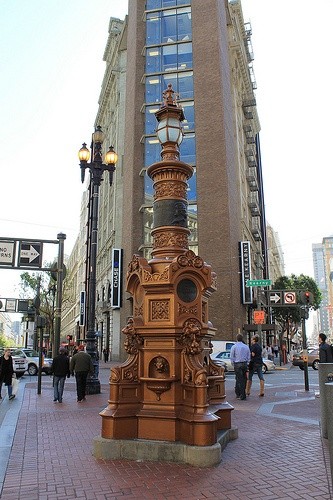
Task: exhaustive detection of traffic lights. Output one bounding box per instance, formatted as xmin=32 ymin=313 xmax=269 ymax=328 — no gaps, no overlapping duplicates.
xmin=28 ymin=308 xmax=35 ymax=322
xmin=305 ymin=292 xmax=311 ymax=306
xmin=66 ymin=335 xmax=72 ymax=340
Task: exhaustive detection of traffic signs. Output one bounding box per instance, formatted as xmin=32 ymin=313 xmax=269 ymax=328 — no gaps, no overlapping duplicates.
xmin=0 ymin=237 xmax=43 ymax=271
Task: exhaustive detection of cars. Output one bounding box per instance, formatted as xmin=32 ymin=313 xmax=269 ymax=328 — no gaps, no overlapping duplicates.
xmin=210 ymin=350 xmax=276 ymax=375
xmin=211 ymin=359 xmax=228 ymax=373
xmin=292 ymin=347 xmax=320 ymax=371
xmin=14 ymin=349 xmax=54 ymax=376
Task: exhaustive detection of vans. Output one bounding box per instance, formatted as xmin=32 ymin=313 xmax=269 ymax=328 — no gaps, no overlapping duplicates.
xmin=210 ymin=340 xmax=237 ymax=354
xmin=0 ymin=347 xmax=29 ymax=378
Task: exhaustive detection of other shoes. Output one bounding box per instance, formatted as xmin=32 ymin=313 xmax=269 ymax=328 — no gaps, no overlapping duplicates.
xmin=77 ymin=401 xmax=81 ymax=402
xmin=259 ymin=394 xmax=264 ymax=397
xmin=54 ymin=398 xmax=58 ymax=401
xmin=9 ymin=395 xmax=15 ymax=400
xmin=236 ymin=396 xmax=241 ymax=400
xmin=246 ymin=393 xmax=250 ymax=396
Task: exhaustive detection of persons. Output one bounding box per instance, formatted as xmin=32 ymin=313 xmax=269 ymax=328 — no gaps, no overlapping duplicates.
xmin=275 ymin=345 xmax=279 ymax=358
xmin=0 ymin=349 xmax=15 ymax=400
xmin=103 ymin=348 xmax=108 ymax=363
xmin=50 ymin=347 xmax=70 ymax=403
xmin=234 ymin=336 xmax=265 ymax=397
xmin=262 ymin=344 xmax=271 ymax=358
xmin=74 ymin=348 xmax=77 ymax=354
xmin=317 ymin=333 xmax=333 ymax=398
xmin=70 ymin=345 xmax=94 ymax=403
xmin=230 ymin=334 xmax=250 ymax=400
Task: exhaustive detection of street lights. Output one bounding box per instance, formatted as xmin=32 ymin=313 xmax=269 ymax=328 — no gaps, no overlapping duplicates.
xmin=73 ymin=124 xmax=119 ymax=395
